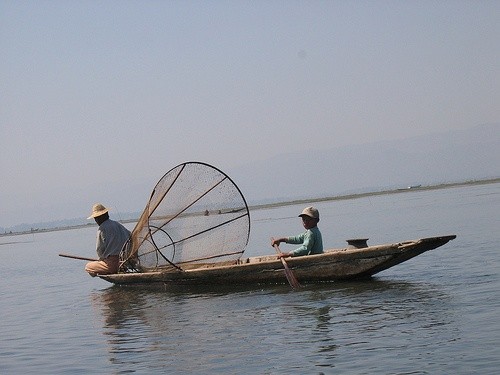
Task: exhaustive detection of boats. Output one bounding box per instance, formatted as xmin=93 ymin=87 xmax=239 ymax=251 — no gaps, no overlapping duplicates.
xmin=95 ymin=234 xmax=458 ymax=287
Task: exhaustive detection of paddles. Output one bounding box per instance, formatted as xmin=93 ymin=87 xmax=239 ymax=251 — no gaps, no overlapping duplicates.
xmin=58 ymin=254 xmax=99 ymax=261
xmin=270 ymin=237 xmax=300 ymax=290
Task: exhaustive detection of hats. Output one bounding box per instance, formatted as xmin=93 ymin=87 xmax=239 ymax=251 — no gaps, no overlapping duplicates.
xmin=86 ymin=203 xmax=113 ymax=219
xmin=298 ymin=206 xmax=319 ymax=218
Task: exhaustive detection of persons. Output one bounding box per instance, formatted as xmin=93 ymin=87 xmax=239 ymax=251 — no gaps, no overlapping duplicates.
xmin=85 ymin=203 xmax=132 ymax=278
xmin=272 ymin=206 xmax=323 ymax=260
